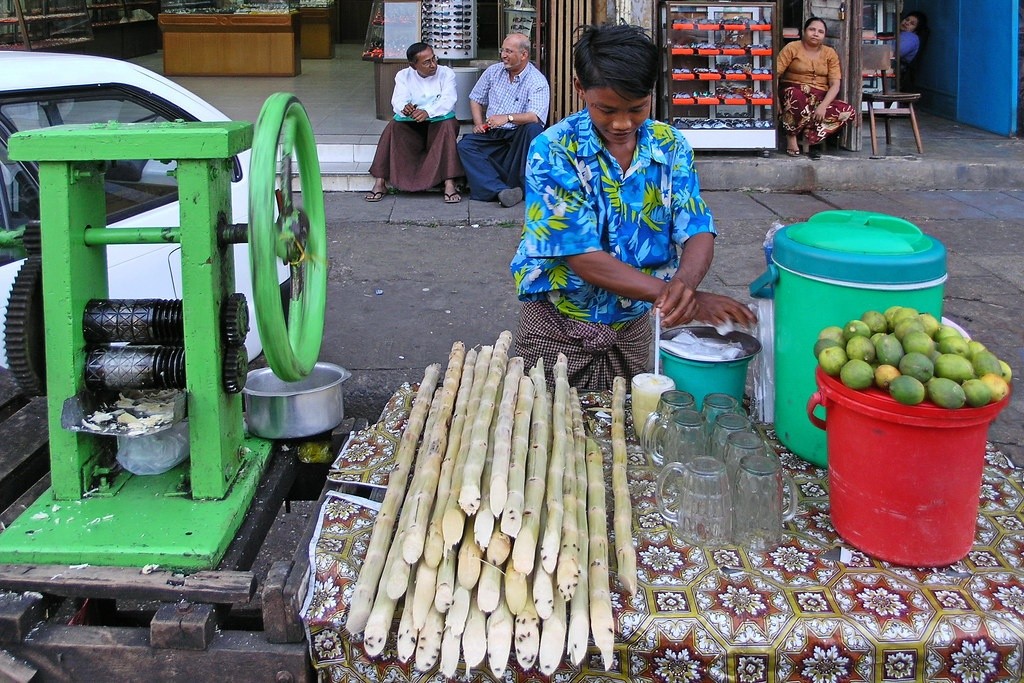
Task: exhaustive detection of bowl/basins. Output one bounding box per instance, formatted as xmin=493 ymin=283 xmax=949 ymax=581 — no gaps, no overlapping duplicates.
xmin=242 ymin=362 xmax=352 ymax=438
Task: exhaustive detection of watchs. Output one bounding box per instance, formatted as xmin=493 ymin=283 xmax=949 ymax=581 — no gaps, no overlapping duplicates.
xmin=507 ymin=114 xmax=514 ymax=123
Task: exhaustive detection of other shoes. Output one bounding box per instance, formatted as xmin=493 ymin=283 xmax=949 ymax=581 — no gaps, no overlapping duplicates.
xmin=498 ymin=187 xmax=523 ymax=207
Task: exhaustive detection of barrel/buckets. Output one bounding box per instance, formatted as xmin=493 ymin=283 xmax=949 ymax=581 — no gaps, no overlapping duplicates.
xmin=806 ymin=363 xmax=1012 ymax=568
xmin=658 ymin=349 xmax=755 ymax=414
xmin=748 ymin=210 xmax=972 ymax=468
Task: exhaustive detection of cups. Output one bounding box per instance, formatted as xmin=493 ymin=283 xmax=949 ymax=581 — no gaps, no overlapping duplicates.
xmin=654 ymin=456 xmax=732 ymax=547
xmin=732 ymin=455 xmax=798 ymax=553
xmin=712 ymin=415 xmax=754 ymax=461
xmin=632 ymin=373 xmax=676 ymax=440
xmin=721 ymin=431 xmax=775 ymax=483
xmin=651 ymin=410 xmax=708 ymax=477
xmin=639 ymin=391 xmax=697 ymax=455
xmin=701 ymin=392 xmax=739 ymax=425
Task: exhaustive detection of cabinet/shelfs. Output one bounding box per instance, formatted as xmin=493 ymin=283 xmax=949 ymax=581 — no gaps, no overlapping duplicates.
xmin=362 ymin=0 xmax=422 ymax=120
xmin=0 ymin=0 xmax=98 ymax=55
xmin=658 ymin=0 xmax=783 ymax=158
xmin=9 ymin=0 xmax=162 ymax=59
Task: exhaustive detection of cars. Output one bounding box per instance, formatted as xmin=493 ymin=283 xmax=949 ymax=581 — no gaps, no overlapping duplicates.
xmin=0 ymin=47 xmax=296 ymax=381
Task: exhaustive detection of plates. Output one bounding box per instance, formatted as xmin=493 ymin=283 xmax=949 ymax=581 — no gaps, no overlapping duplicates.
xmin=660 ymin=326 xmax=762 ymax=362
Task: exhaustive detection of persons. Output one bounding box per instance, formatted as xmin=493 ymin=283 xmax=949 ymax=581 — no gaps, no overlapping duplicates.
xmin=364 ymin=43 xmax=464 ymax=203
xmin=888 ymin=13 xmax=920 ymax=90
xmin=457 ymin=33 xmax=550 ymax=207
xmin=510 ymin=22 xmax=758 ymax=393
xmin=119 ymin=6 xmax=155 ymax=23
xmin=777 ymin=17 xmax=856 ymax=156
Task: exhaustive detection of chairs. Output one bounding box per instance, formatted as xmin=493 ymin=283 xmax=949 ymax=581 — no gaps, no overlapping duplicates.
xmin=861 ymin=45 xmax=923 ymax=155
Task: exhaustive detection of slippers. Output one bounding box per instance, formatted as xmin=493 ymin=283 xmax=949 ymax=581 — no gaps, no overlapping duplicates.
xmin=365 ymin=191 xmax=388 ymax=201
xmin=445 ymin=192 xmax=462 ymax=202
xmin=786 ymin=148 xmax=800 ymax=157
xmin=804 ymin=151 xmax=820 ymax=159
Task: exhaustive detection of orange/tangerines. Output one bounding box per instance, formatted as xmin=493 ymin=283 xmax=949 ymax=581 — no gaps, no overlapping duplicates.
xmin=813 ymin=305 xmax=1012 ymax=407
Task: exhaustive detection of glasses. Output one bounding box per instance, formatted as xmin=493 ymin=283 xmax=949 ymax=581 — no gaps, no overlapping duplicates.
xmin=510 ymin=17 xmax=532 ymax=31
xmin=420 ymin=0 xmax=473 ymax=50
xmin=498 ymin=47 xmax=525 ymax=56
xmin=670 ymin=17 xmax=774 ymax=130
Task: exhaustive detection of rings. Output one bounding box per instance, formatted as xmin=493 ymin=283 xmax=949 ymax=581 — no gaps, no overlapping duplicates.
xmin=488 ymin=122 xmax=491 ymax=124
